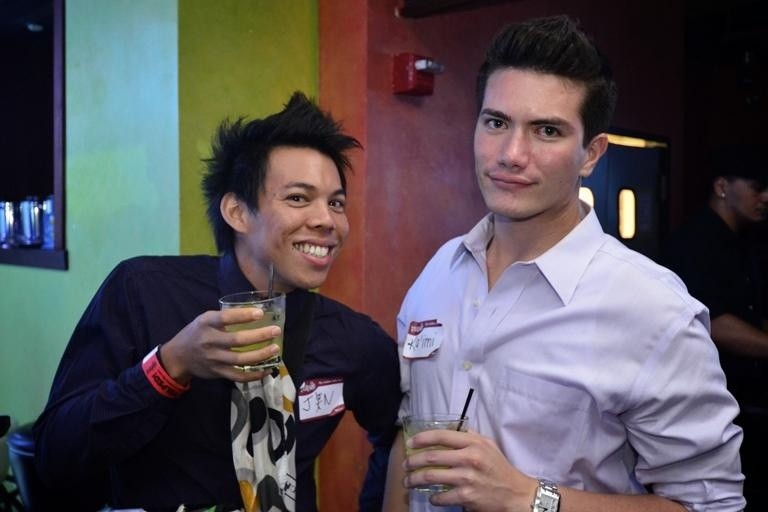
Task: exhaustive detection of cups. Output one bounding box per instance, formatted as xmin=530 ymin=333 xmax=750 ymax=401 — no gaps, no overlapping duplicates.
xmin=401 ymin=412 xmax=470 ymax=495
xmin=218 ymin=290 xmax=286 ymax=373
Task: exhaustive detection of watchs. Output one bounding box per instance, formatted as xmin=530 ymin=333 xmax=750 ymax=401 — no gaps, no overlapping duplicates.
xmin=530 ymin=474 xmax=563 ymax=510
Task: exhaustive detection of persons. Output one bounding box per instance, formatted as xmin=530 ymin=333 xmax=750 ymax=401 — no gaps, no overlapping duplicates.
xmin=654 ymin=139 xmax=768 ymax=511
xmin=28 ymin=89 xmax=404 ymax=512
xmin=381 ymin=14 xmax=749 ymax=511
